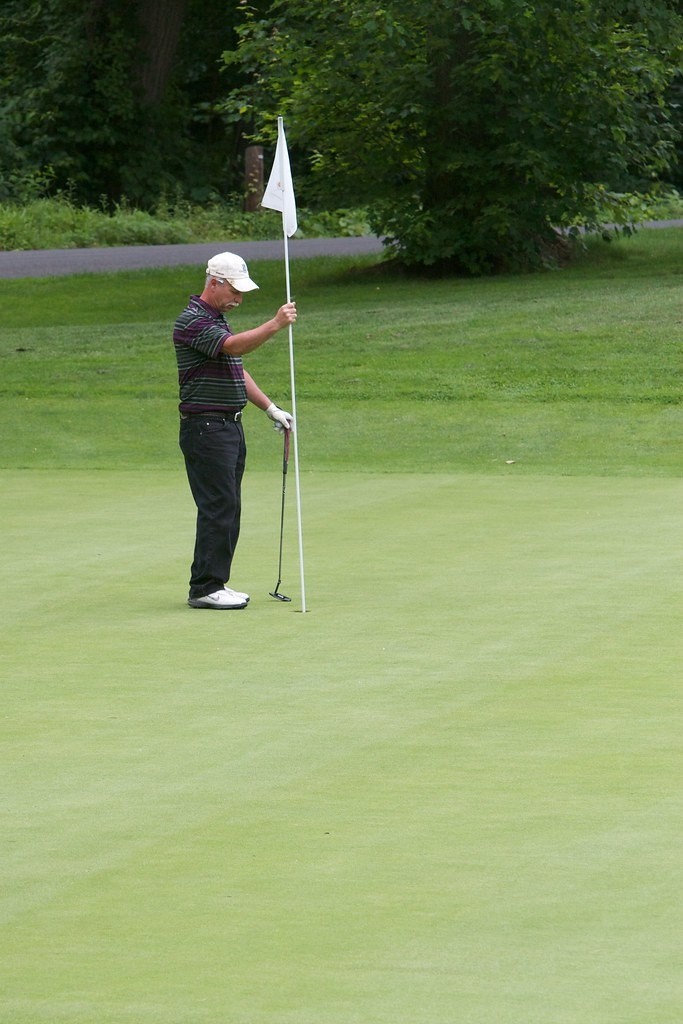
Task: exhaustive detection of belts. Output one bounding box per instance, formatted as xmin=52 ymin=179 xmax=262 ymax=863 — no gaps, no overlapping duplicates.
xmin=182 ymin=410 xmax=243 ymax=422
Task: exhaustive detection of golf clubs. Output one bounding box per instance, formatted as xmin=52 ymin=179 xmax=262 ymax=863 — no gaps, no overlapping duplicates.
xmin=268 ymin=418 xmax=292 ymax=603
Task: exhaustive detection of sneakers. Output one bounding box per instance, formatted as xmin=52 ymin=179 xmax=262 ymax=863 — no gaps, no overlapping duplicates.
xmin=188 ymin=586 xmax=249 ymax=609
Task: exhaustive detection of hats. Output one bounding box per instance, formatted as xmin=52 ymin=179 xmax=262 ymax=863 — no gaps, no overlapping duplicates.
xmin=206 ymin=252 xmax=259 ymax=293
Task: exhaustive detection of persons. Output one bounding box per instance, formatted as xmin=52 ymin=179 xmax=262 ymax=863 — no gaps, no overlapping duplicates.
xmin=172 ymin=251 xmax=297 ymax=609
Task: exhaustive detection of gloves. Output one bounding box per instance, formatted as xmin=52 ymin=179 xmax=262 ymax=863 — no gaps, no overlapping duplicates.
xmin=264 ymin=403 xmax=293 ymax=434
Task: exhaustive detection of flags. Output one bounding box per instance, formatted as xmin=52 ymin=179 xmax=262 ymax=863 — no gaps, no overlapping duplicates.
xmin=261 ymin=123 xmax=298 ymax=237
